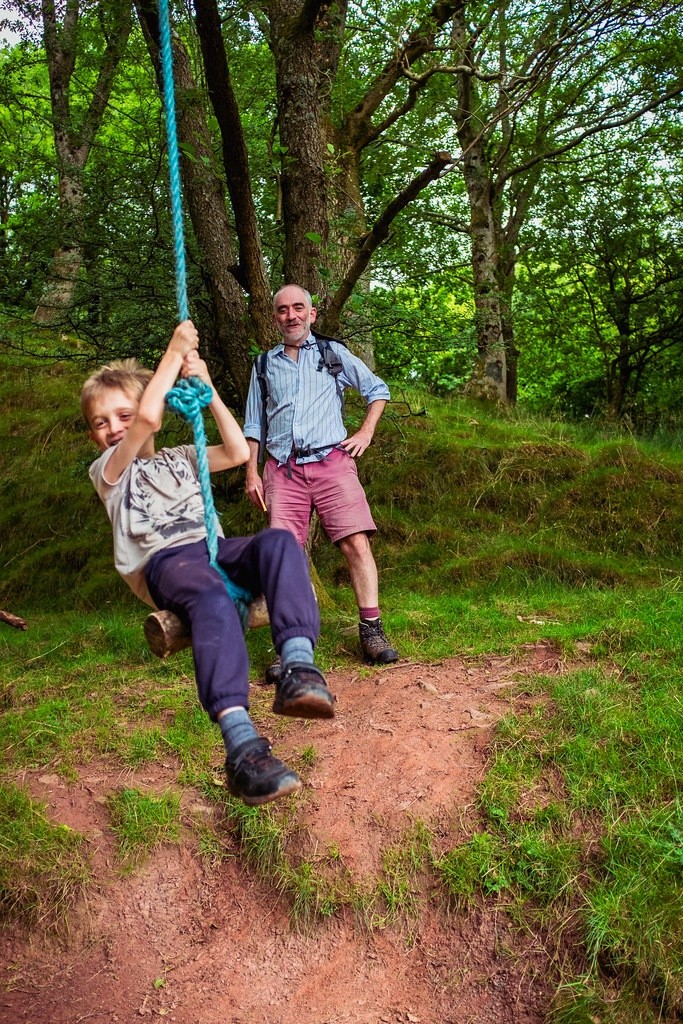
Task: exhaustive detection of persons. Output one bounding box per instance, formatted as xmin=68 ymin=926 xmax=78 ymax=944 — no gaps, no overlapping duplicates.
xmin=242 ymin=284 xmax=399 ymax=681
xmin=82 ymin=320 xmax=335 ymax=806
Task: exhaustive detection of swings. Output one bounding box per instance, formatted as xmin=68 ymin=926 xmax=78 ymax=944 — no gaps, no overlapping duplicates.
xmin=141 ymin=0 xmax=317 ymax=657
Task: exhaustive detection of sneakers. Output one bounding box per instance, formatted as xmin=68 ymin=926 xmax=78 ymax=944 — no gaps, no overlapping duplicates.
xmin=273 ymin=662 xmax=334 ymax=718
xmin=266 ymin=656 xmax=281 ymax=684
xmin=359 ymin=618 xmax=398 ymax=665
xmin=225 ymin=737 xmax=301 ymax=805
